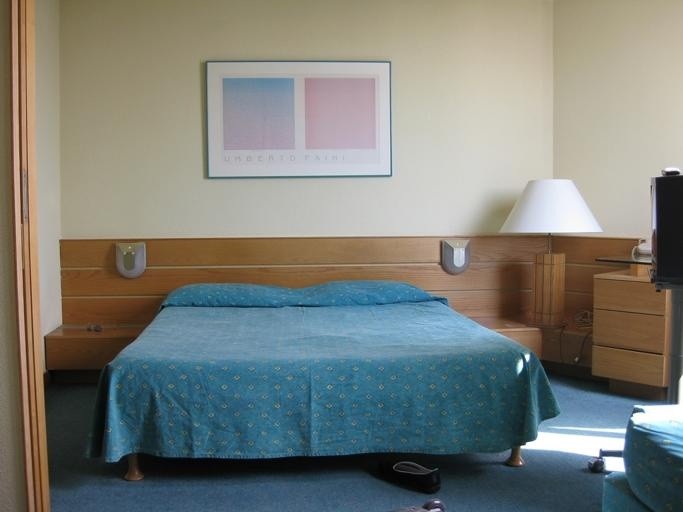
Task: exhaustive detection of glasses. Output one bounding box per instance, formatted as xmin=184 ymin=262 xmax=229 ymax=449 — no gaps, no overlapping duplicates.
xmin=114 ymin=242 xmax=146 ymax=279
xmin=441 ymin=238 xmax=471 ymax=275
xmin=499 ymin=179 xmax=603 ymax=330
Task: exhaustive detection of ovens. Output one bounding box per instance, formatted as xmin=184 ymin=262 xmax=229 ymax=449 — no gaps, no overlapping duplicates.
xmin=83 ymin=301 xmax=560 ymax=482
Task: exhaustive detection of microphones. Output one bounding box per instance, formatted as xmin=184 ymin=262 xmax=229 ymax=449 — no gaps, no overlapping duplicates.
xmin=600 ymin=402 xmax=683 ymax=512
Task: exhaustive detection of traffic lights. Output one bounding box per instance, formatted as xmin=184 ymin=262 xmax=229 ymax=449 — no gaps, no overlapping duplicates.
xmin=159 ymin=277 xmax=448 ymax=307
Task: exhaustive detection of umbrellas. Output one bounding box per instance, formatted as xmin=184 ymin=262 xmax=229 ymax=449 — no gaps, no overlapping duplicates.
xmin=380 ymin=461 xmax=440 ymax=493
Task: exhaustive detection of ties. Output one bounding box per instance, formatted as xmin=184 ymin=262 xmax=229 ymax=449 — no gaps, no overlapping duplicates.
xmin=650 ymin=174 xmax=683 ymax=292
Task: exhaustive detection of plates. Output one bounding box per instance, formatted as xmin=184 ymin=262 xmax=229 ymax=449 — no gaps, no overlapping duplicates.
xmin=591 ymin=268 xmax=673 ymax=401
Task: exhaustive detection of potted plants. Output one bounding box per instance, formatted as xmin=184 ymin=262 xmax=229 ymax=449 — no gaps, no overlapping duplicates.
xmin=205 ymin=60 xmax=393 ymax=179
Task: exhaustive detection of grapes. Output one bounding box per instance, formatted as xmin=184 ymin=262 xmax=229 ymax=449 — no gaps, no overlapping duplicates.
xmin=46 ymin=324 xmax=144 ymax=370
xmin=469 ymin=316 xmax=543 ymax=362
